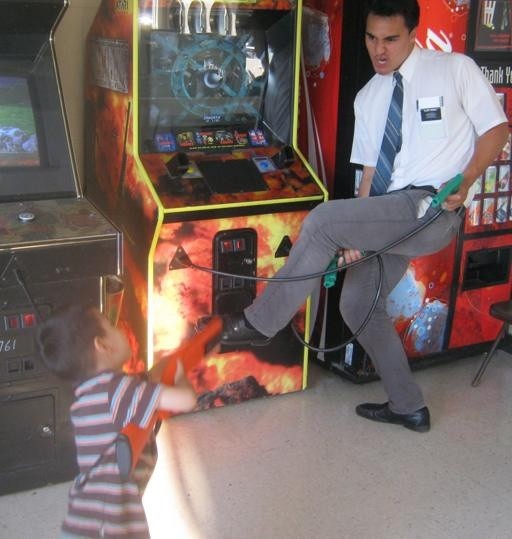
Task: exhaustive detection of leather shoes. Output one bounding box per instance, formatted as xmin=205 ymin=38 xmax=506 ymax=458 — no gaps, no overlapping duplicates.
xmin=354 ymin=397 xmax=431 ymax=433
xmin=194 ymin=312 xmax=271 ymax=347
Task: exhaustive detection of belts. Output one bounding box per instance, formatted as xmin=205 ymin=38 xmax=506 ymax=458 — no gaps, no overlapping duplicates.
xmin=398 ymin=184 xmax=468 ymax=221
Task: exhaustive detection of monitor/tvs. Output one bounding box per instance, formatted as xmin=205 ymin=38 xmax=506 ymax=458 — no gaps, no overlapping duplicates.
xmin=148 ymin=29 xmax=268 ymax=128
xmin=0 ymin=76 xmax=40 ymax=167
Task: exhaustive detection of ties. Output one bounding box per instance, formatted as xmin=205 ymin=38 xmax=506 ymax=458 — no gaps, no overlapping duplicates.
xmin=368 ymin=69 xmax=405 ymax=197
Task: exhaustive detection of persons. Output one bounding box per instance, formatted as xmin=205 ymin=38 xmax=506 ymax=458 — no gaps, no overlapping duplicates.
xmin=34 ymin=300 xmax=198 ymax=539
xmin=220 ymin=0 xmax=510 ymax=431
